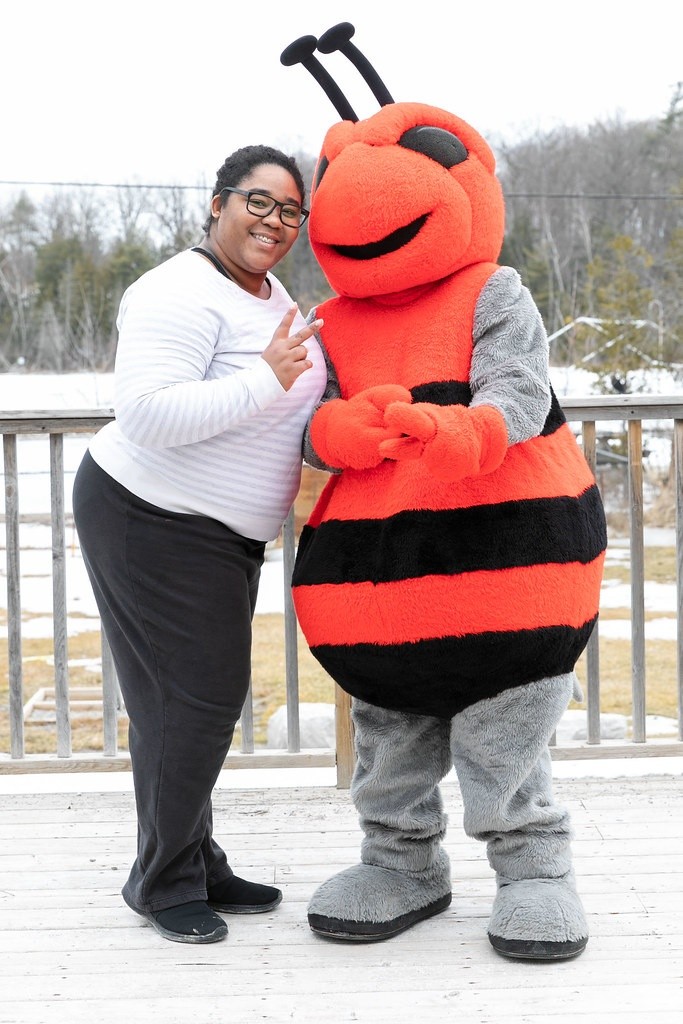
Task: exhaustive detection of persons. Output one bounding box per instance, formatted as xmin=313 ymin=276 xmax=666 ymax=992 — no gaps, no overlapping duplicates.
xmin=75 ymin=146 xmax=328 ymax=946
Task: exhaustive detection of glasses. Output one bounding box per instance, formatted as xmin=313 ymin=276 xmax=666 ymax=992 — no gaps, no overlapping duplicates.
xmin=216 ymin=186 xmax=310 ymax=228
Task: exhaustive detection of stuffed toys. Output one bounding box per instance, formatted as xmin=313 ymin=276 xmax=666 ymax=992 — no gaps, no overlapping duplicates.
xmin=277 ymin=19 xmax=607 ymax=964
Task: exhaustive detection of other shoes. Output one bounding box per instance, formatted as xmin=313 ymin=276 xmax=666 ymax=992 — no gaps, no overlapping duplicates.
xmin=205 ymin=875 xmax=283 ymax=914
xmin=146 ymin=900 xmax=229 ymax=945
